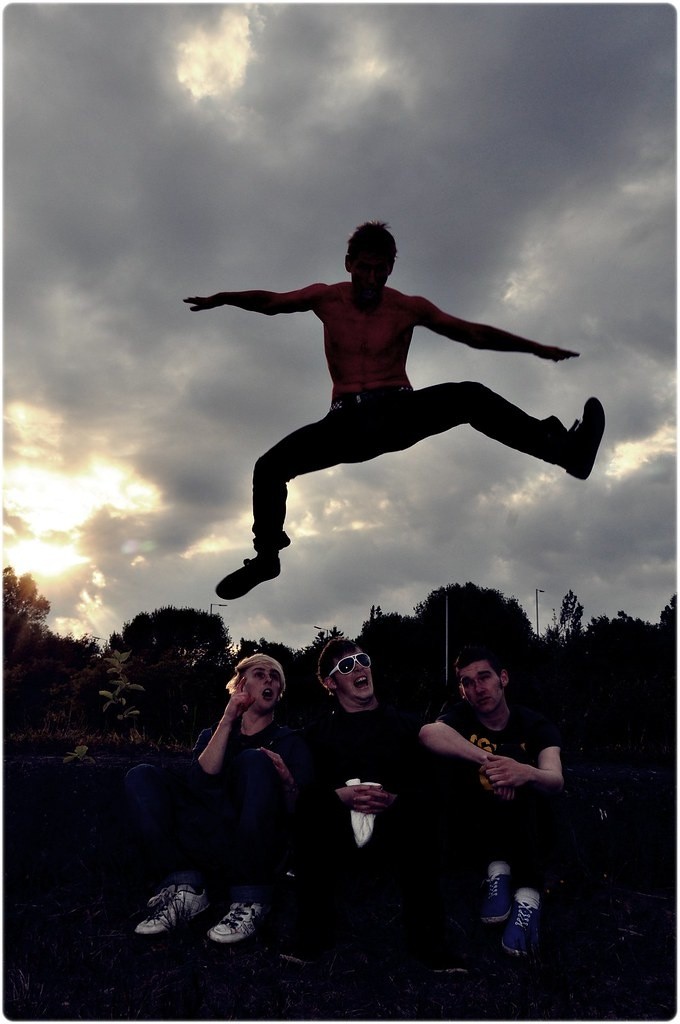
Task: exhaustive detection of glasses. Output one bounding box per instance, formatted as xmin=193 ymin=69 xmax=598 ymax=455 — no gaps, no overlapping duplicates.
xmin=329 ymin=654 xmax=372 ymax=677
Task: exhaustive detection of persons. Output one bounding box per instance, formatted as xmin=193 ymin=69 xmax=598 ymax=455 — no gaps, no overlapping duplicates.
xmin=181 ymin=221 xmax=604 ymax=600
xmin=123 ymin=638 xmax=575 ymax=956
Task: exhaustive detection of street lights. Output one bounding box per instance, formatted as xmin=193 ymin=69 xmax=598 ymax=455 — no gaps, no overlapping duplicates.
xmin=209 ymin=603 xmax=227 ymax=615
xmin=315 ymin=626 xmax=329 ymax=637
xmin=536 ymin=588 xmax=544 ymax=635
xmin=432 ymin=589 xmax=449 ymax=687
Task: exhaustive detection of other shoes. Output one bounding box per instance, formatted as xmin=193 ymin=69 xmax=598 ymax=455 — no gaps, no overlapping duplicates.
xmin=217 ymin=555 xmax=281 ymax=600
xmin=280 ymin=934 xmax=356 ymax=966
xmin=400 ymin=916 xmax=452 ymax=973
xmin=562 ymin=397 xmax=606 ymax=479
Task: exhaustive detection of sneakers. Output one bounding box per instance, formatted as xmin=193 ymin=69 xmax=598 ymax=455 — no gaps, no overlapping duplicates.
xmin=135 ymin=885 xmax=208 ymax=935
xmin=481 ymin=873 xmax=512 ymax=924
xmin=502 ymin=899 xmax=541 ymax=956
xmin=207 ymin=901 xmax=272 ymax=944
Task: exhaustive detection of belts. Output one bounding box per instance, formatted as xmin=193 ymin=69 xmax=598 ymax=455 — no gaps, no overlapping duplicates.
xmin=330 ymin=387 xmax=413 ymax=411
